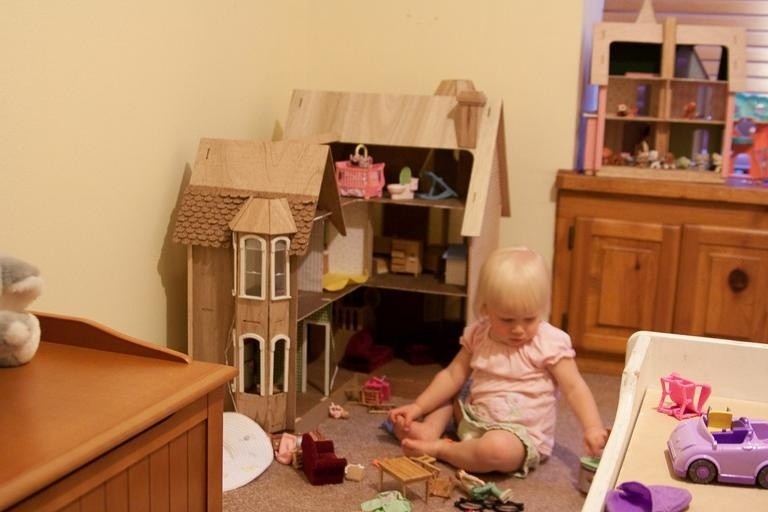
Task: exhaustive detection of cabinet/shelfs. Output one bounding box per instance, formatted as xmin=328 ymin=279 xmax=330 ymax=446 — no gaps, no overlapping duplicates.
xmin=549 ymin=169 xmax=768 ymax=376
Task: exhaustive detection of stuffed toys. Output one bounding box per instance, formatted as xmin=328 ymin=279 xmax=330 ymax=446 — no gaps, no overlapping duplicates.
xmin=0 ymin=253 xmax=47 ymax=372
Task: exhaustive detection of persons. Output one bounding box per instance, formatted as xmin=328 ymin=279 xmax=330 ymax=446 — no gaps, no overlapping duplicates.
xmin=387 ymin=243 xmax=606 ymax=478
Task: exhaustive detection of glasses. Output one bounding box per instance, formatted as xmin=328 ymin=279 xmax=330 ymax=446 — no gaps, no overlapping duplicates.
xmin=452 ymin=495 xmax=527 ymax=512
